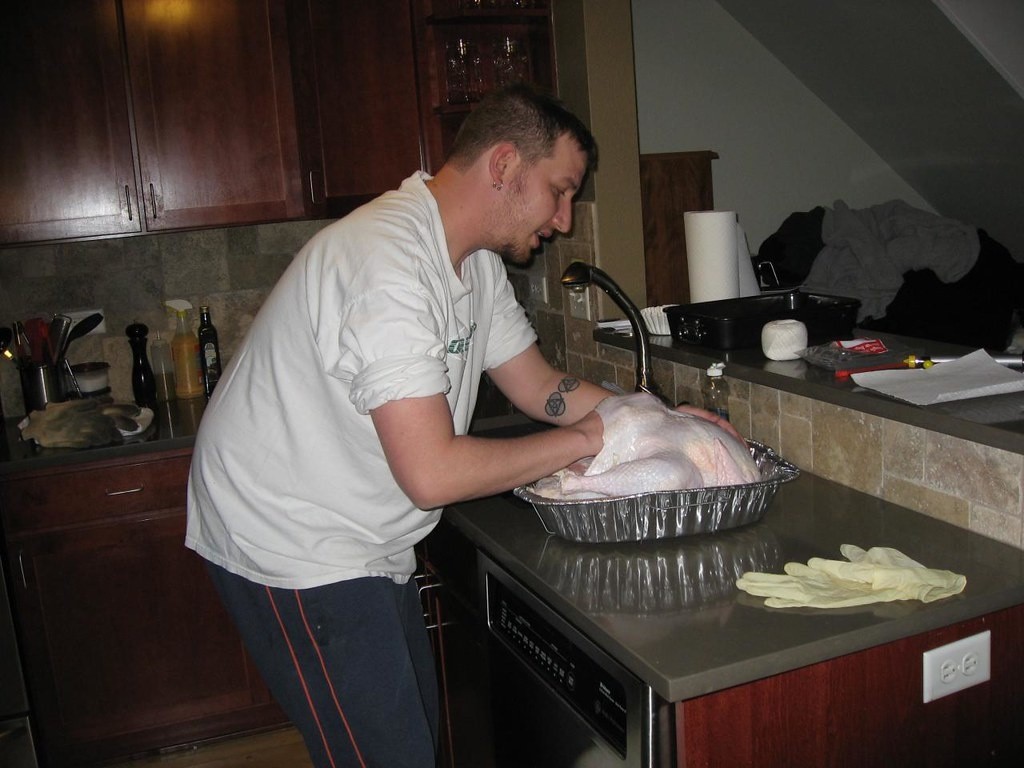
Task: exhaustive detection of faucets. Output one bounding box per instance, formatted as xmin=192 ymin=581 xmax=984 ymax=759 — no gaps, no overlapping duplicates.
xmin=560 ymin=260 xmax=654 ymax=394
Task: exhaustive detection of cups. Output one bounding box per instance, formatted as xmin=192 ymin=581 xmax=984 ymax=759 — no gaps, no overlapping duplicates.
xmin=14 ymin=362 xmax=62 ymax=416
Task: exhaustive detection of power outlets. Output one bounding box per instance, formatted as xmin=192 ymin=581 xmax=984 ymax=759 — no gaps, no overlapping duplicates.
xmin=62 ymin=307 xmax=106 ymax=337
xmin=568 ymin=287 xmax=592 ymax=321
xmin=922 ymin=630 xmax=992 ymax=704
xmin=529 ymin=275 xmax=550 ymax=305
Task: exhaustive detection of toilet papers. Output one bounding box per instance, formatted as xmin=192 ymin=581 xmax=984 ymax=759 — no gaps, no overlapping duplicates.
xmin=682 ymin=207 xmax=766 ymax=303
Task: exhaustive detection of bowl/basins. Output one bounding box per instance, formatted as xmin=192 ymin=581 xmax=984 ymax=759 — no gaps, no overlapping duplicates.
xmin=640 ymin=304 xmax=680 ymax=335
xmin=64 ymin=361 xmax=115 ymax=410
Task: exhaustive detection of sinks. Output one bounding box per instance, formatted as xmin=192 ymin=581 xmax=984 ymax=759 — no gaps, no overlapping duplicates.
xmin=474 ymin=420 xmax=558 ymax=510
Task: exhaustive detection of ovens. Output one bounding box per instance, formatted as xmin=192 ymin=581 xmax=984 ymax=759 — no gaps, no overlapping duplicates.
xmin=417 ymin=528 xmax=675 ymax=768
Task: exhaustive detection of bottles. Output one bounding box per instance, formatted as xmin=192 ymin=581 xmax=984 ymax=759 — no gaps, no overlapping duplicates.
xmin=150 ymin=329 xmax=175 ymax=399
xmin=171 ymin=309 xmax=205 ymax=399
xmin=197 ymin=306 xmax=223 ymax=396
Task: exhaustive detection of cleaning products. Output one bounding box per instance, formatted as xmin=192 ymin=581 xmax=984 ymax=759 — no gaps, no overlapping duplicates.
xmin=162 ymin=297 xmax=205 ymax=399
xmin=701 ymin=362 xmax=730 ymax=421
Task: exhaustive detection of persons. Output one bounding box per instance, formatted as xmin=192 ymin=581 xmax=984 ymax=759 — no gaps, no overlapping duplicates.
xmin=185 ymin=86 xmax=749 ymax=768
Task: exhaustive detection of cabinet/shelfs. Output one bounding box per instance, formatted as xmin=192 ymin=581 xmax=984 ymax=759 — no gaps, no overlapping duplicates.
xmin=0 ymin=0 xmax=313 ymax=248
xmin=289 ymin=1 xmax=425 ymax=221
xmin=410 ymin=0 xmax=559 ymax=175
xmin=0 ymin=447 xmax=295 ymax=768
